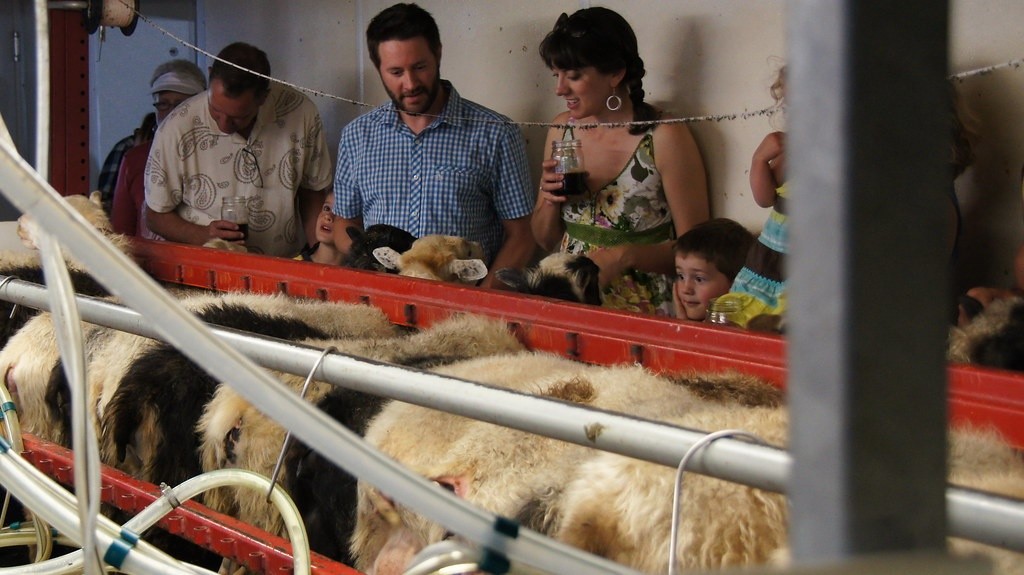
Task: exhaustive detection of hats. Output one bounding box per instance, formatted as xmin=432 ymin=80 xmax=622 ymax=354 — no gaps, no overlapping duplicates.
xmin=149 ymin=59 xmax=207 ymax=95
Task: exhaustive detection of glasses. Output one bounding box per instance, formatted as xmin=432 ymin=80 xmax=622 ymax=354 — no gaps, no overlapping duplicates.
xmin=152 ymin=100 xmax=180 ymax=111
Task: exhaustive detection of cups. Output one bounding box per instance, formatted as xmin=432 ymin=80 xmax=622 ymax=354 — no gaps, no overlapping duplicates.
xmin=550 ymin=140 xmax=586 ymax=197
xmin=221 ymin=196 xmax=249 ymax=242
xmin=709 ymin=295 xmax=747 ymax=330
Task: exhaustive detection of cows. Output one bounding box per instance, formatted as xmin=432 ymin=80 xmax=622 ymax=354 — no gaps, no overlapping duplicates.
xmin=0 ymin=189 xmax=1024 ymax=575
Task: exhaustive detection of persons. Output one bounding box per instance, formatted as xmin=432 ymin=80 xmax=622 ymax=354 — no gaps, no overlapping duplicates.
xmin=715 ymin=64 xmax=788 ymax=333
xmin=142 ymin=40 xmax=337 ymax=267
xmin=330 ymin=2 xmax=534 ymax=291
xmin=530 ymin=8 xmax=711 ymax=291
xmin=670 ymin=217 xmax=749 ymax=323
xmin=946 ymin=81 xmax=1024 ymax=372
xmin=95 ymin=58 xmax=211 ymax=244
